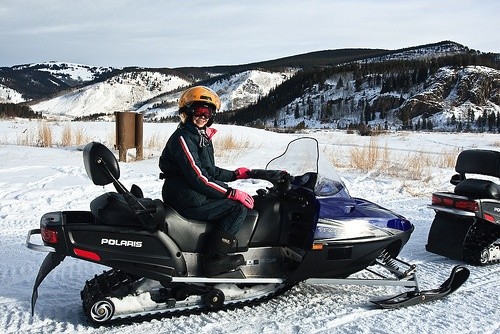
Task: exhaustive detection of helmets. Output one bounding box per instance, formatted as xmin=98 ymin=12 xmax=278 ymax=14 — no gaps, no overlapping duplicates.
xmin=178 ymin=85 xmax=221 ymax=111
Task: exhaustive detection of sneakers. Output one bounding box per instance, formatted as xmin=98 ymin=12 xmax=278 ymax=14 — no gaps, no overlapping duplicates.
xmin=200 ymin=254 xmax=244 ymax=277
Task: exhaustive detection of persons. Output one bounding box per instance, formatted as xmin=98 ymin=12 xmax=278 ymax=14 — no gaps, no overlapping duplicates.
xmin=158 ymin=85 xmax=255 ymax=277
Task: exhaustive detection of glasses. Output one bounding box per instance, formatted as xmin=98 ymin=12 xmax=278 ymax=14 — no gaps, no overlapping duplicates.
xmin=190 ymin=105 xmax=212 ymax=118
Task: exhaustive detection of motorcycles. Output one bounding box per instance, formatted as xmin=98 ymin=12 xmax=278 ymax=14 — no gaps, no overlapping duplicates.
xmin=25 ymin=136 xmax=470 ymax=326
xmin=425 ymin=148 xmax=500 ymax=265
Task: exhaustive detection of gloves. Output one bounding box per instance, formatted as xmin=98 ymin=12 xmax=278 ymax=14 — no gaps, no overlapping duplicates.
xmin=234 ymin=167 xmax=251 ymax=179
xmin=226 ymin=188 xmax=254 ymax=210
